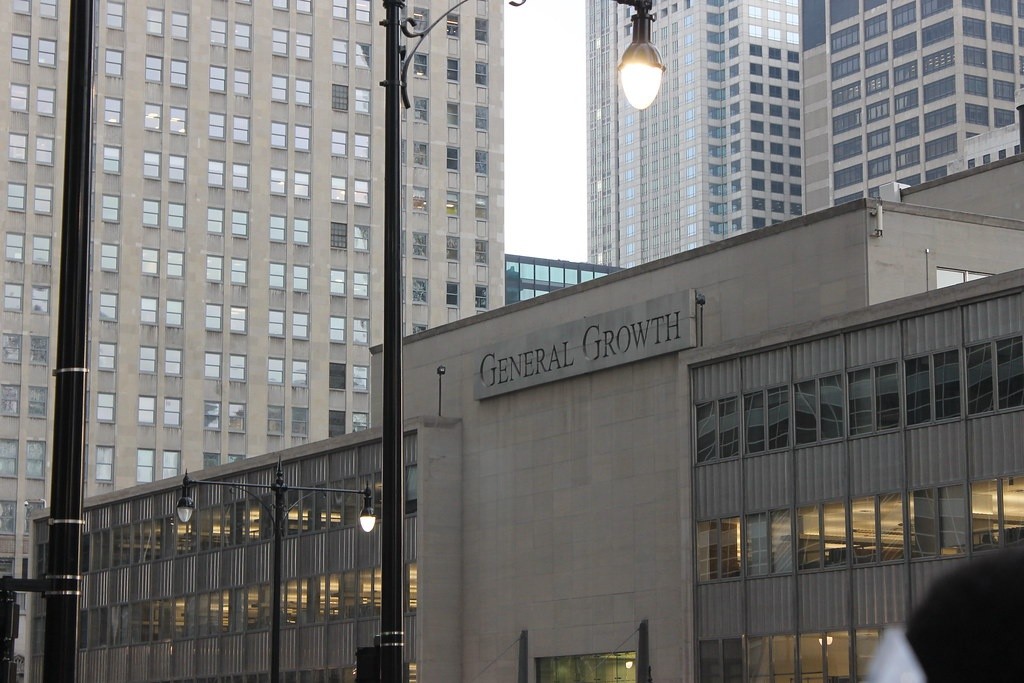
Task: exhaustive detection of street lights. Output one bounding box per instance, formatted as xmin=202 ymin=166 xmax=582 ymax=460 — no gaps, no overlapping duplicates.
xmin=382 ymin=2 xmax=666 ymax=680
xmin=176 ymin=475 xmax=376 ymax=681
